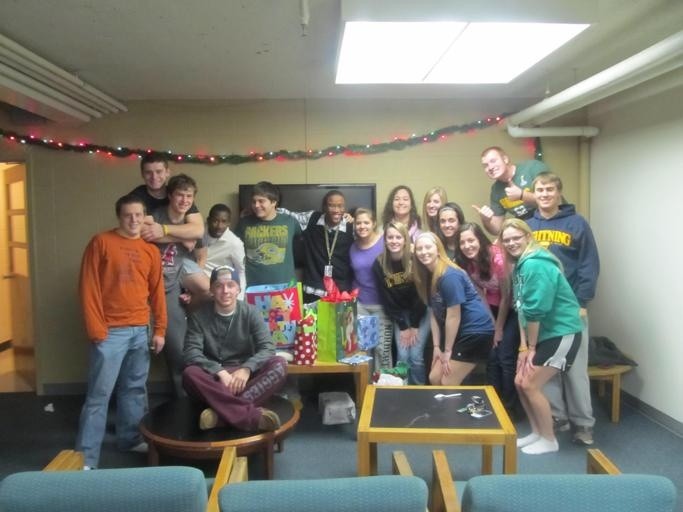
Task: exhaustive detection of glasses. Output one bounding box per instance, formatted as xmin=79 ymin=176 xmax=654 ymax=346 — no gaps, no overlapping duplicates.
xmin=502 ymin=234 xmax=527 ymax=243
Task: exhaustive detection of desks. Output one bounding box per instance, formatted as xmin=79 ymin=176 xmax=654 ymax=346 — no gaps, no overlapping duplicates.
xmin=357 ymin=384 xmax=514 ymax=476
xmin=280 ymin=349 xmax=370 ymax=408
xmin=139 ymin=394 xmax=299 ymax=479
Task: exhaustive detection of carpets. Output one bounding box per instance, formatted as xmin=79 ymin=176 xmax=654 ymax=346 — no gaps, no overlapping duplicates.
xmin=0 ymin=390 xmax=683 ymax=512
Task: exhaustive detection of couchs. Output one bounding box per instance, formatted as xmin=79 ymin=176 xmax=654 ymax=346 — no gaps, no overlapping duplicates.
xmin=432 ymin=449 xmax=677 ymax=512
xmin=217 ymin=451 xmax=429 ymax=512
xmin=0 ymin=442 xmax=236 ymax=511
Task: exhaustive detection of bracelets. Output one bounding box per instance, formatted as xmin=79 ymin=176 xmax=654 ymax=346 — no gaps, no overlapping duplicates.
xmin=434 ymin=344 xmax=439 ymax=347
xmin=529 ymin=345 xmax=535 ymax=350
xmin=163 ymin=224 xmax=168 ymax=235
xmin=520 ymin=190 xmax=524 ymax=200
xmin=518 ymin=347 xmax=527 ymax=352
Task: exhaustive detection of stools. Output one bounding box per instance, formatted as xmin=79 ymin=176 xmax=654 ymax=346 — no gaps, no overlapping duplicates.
xmin=584 ymin=363 xmax=633 ymax=422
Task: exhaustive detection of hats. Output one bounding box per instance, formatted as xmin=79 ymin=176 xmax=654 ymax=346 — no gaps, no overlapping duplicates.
xmin=209 ymin=265 xmax=240 ymax=287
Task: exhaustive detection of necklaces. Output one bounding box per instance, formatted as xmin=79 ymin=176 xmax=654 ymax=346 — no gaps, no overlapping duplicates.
xmin=213 ymin=305 xmax=238 ymax=360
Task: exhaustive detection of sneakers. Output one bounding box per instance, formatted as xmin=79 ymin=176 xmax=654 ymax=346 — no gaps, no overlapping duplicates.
xmin=128 ymin=443 xmax=148 ymax=452
xmin=552 ymin=419 xmax=570 ymax=433
xmin=199 ymin=408 xmax=224 ymax=430
xmin=256 ymin=407 xmax=280 ymax=432
xmin=521 ymin=437 xmax=559 ymax=455
xmin=570 ymin=424 xmax=594 ymax=445
xmin=516 ymin=432 xmax=540 ymax=448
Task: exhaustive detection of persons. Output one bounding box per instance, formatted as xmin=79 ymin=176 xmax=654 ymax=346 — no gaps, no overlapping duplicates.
xmin=435 ymin=202 xmax=465 ymax=266
xmin=499 ymin=218 xmax=586 ymax=457
xmin=454 ymin=222 xmax=520 ymax=423
xmin=76 ymin=196 xmax=167 ymax=471
xmin=473 ymin=146 xmax=568 ymax=238
xmin=423 ymin=187 xmax=448 ymax=231
xmin=130 ymin=152 xmax=212 ymax=310
xmin=293 ymin=191 xmax=356 ymax=292
xmin=181 ymin=266 xmax=288 ymax=429
xmin=413 ymin=231 xmax=495 ymax=386
xmin=143 ymin=173 xmax=211 ymax=371
xmin=523 ymin=172 xmax=600 ymax=446
xmin=372 ymin=222 xmax=432 ymax=386
xmin=383 ymin=185 xmax=422 ymax=252
xmin=348 ymin=208 xmax=394 ymax=371
xmin=204 ymin=203 xmax=247 ymax=302
xmin=236 ymin=181 xmax=306 ymax=283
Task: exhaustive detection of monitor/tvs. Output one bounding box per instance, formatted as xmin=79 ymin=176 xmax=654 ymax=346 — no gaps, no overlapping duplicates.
xmin=239 ymin=183 xmax=377 ymax=221
